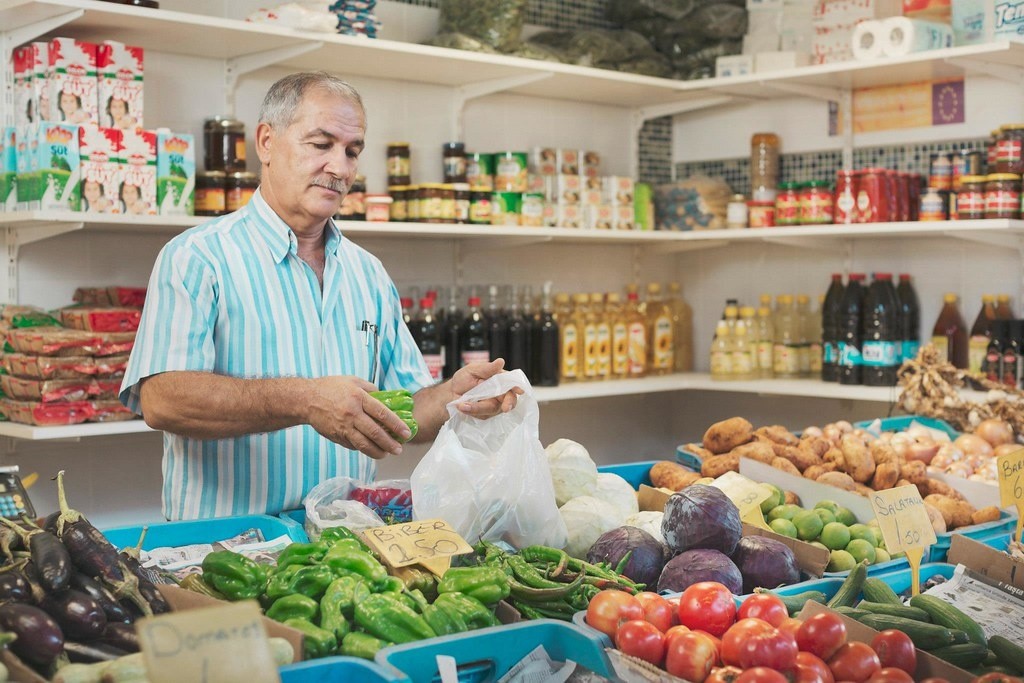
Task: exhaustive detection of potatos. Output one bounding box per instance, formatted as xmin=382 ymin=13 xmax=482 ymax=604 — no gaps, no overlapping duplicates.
xmin=648 ymin=418 xmax=1001 ymax=535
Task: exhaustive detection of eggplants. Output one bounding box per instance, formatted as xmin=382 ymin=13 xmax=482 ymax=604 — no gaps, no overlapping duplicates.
xmin=0 ymin=470 xmax=173 ymax=677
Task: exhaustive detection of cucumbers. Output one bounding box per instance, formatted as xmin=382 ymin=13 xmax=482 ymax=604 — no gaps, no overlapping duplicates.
xmin=753 ymin=557 xmax=1024 ymax=677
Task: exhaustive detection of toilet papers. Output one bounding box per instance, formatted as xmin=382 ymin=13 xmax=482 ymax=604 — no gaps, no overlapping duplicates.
xmin=851 ymin=15 xmax=955 ymax=63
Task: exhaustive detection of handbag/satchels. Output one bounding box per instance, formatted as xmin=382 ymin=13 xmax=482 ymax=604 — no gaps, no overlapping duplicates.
xmin=409 ymin=370 xmax=570 ymax=553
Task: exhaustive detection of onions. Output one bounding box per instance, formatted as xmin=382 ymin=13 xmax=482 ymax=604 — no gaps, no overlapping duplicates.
xmin=801 ymin=417 xmax=1024 ymax=484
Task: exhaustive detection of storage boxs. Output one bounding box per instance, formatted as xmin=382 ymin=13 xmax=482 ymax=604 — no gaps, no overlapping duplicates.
xmin=29 ymin=42 xmax=49 ymax=123
xmin=79 ymin=125 xmax=119 ymax=212
xmin=15 ymin=124 xmax=29 ymax=210
xmin=97 ymin=40 xmax=144 ymax=130
xmin=101 ymin=415 xmax=1024 ymax=683
xmin=13 ymin=47 xmax=32 ymax=124
xmin=48 ymin=37 xmax=98 ymax=129
xmin=0 ymin=127 xmax=16 ymax=212
xmin=156 ymin=128 xmax=196 ymax=216
xmin=28 ymin=121 xmax=81 ymax=211
xmin=118 ymin=130 xmax=156 ymax=216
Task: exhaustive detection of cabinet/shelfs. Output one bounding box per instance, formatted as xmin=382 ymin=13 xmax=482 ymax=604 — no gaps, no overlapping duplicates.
xmin=0 ymin=0 xmax=1024 ymax=440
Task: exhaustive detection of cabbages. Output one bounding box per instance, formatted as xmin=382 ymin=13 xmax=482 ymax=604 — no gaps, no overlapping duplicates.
xmin=540 ymin=437 xmax=667 ymax=562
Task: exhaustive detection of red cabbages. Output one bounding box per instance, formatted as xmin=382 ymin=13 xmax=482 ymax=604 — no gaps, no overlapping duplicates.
xmin=584 ymin=484 xmax=800 ymax=595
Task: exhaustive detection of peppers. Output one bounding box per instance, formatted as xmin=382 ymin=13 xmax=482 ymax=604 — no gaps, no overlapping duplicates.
xmin=362 ymin=389 xmax=419 ymax=445
xmin=160 ymin=525 xmax=647 ymax=664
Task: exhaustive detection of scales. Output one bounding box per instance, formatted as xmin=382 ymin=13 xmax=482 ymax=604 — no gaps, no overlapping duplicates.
xmin=0 ymin=465 xmax=37 ymax=522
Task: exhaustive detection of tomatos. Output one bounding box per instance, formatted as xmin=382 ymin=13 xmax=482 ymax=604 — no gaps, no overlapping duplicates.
xmin=586 ymin=581 xmax=1024 ymax=683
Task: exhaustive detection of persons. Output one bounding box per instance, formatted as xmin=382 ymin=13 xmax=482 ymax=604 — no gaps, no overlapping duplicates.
xmin=106 ymin=94 xmax=136 ymax=130
xmin=38 ymin=92 xmax=52 ymax=119
xmin=117 ymin=73 xmax=525 ymax=523
xmin=120 ymin=181 xmax=151 ymax=215
xmin=57 ymin=89 xmax=91 ymax=125
xmin=79 ymin=178 xmax=115 ymax=212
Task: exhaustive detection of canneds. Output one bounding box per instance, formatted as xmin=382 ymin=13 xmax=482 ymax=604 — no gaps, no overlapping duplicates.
xmin=772 ymin=123 xmax=1024 ymax=226
xmin=331 ymin=174 xmax=367 ymax=222
xmin=194 ymin=119 xmax=257 ymax=216
xmin=386 ymin=142 xmax=472 ymax=224
xmin=726 ymin=193 xmax=775 ymax=229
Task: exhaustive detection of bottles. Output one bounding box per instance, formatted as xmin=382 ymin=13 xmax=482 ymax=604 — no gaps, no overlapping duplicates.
xmin=711 ymin=273 xmax=923 ymax=384
xmin=400 ymin=282 xmax=692 ymax=386
xmin=932 ymin=294 xmax=1024 ymax=389
